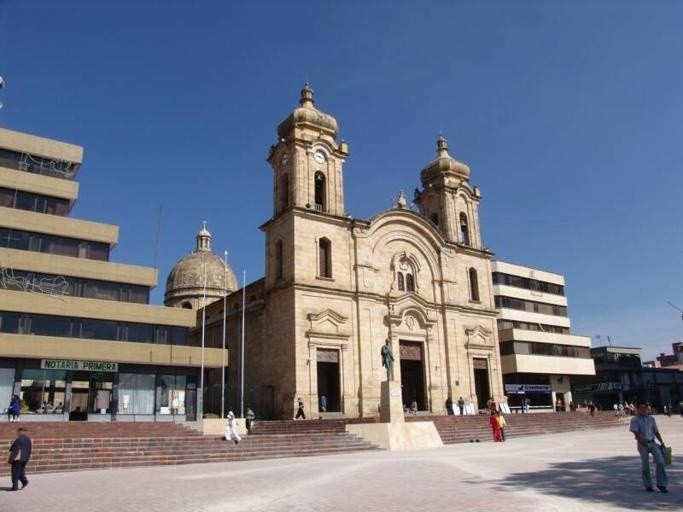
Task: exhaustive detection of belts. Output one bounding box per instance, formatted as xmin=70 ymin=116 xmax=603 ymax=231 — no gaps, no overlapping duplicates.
xmin=647 ymin=440 xmax=654 ymax=443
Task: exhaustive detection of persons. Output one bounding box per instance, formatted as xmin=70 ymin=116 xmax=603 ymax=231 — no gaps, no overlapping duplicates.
xmin=629 ymin=401 xmax=670 ymax=493
xmin=613 ymin=400 xmax=683 ymax=417
xmin=55 ymin=401 xmax=62 ymax=409
xmin=489 ymin=398 xmax=496 ymax=411
xmin=458 ymin=397 xmax=464 ymax=416
xmin=245 ymin=405 xmax=255 ymax=431
xmin=8 ymin=395 xmax=21 ymax=422
xmin=557 ymin=398 xmax=562 ymax=415
xmin=445 ymin=397 xmax=454 ymax=415
xmin=568 ymin=401 xmax=574 ymax=411
xmin=495 ymin=410 xmax=507 ymax=442
xmin=411 ymin=399 xmax=417 ymax=416
xmin=319 ymin=393 xmax=327 ymax=412
xmin=5 ymin=394 xmax=16 ymax=423
xmin=379 ymin=336 xmax=396 ymax=381
xmin=6 ymin=427 xmax=31 ymax=490
xmin=291 ymin=397 xmax=307 ymax=420
xmin=225 ymin=406 xmax=242 ymax=442
xmin=488 ymin=411 xmax=504 ymax=442
xmin=45 ymin=400 xmax=52 ymax=408
xmin=523 ymin=397 xmax=532 ymax=413
xmin=68 ymin=406 xmax=80 ymax=421
xmin=587 ymin=402 xmax=595 ymax=416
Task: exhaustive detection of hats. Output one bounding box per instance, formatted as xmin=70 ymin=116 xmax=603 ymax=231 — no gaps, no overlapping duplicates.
xmin=15 ymin=427 xmax=28 ymax=432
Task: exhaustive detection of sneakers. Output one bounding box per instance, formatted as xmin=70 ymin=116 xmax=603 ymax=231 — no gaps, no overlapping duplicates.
xmin=20 ymin=480 xmax=29 ymax=489
xmin=656 ymin=486 xmax=668 ymax=494
xmin=645 ymin=487 xmax=653 ymax=492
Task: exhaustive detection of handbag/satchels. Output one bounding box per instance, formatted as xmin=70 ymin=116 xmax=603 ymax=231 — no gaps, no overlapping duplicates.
xmin=7 ymin=448 xmax=22 ymax=464
xmin=659 ymin=443 xmax=672 ymax=466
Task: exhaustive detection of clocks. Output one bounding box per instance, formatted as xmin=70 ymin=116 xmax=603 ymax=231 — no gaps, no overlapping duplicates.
xmin=314 ymin=149 xmax=326 ymax=162
xmin=280 ymin=152 xmax=288 ymax=165
